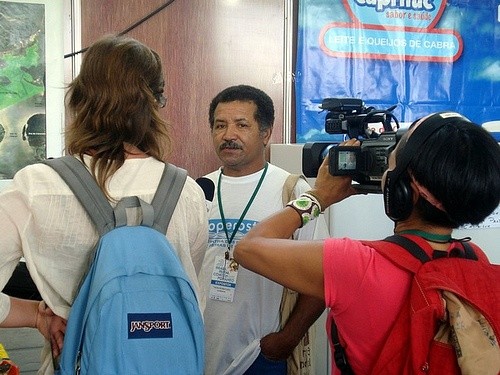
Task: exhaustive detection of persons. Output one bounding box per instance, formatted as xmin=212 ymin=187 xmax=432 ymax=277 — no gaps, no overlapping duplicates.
xmin=194 ymin=84 xmax=327 ymax=375
xmin=0 ymin=34 xmax=210 ymax=375
xmin=234 ymin=112 xmax=500 ymax=375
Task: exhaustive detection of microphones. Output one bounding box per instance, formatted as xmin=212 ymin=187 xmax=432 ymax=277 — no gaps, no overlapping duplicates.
xmin=196 ymin=178 xmax=215 ymax=213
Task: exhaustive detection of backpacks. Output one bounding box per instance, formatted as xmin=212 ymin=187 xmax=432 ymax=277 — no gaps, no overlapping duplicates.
xmin=331 ymin=235 xmax=499 ymax=375
xmin=38 ymin=156 xmax=207 ymax=375
